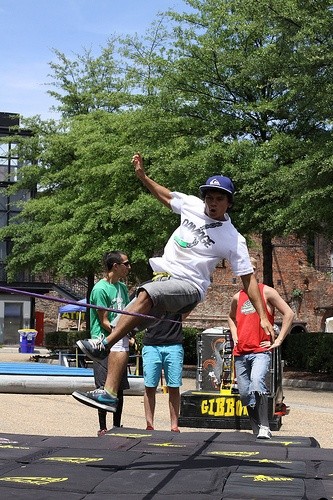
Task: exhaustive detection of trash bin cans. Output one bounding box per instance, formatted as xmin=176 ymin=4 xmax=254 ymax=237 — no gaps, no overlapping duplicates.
xmin=18 ymin=328 xmax=38 ymax=353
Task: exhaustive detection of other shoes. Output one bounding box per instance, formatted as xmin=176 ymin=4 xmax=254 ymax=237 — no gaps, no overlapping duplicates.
xmin=97 ymin=429 xmax=107 ymax=437
xmin=146 ymin=426 xmax=153 ymax=430
xmin=171 ymin=428 xmax=180 ymax=432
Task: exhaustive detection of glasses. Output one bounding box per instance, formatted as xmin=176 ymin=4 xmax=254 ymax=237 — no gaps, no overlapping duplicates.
xmin=113 ymin=260 xmax=130 ymax=266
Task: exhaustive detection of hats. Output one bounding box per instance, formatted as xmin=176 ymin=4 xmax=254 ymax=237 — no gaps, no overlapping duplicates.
xmin=250 ymin=256 xmax=257 ymax=269
xmin=199 ymin=175 xmax=235 ymax=195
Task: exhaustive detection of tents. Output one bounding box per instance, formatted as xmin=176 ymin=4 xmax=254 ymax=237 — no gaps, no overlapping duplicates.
xmin=56 ymin=299 xmax=86 ymax=332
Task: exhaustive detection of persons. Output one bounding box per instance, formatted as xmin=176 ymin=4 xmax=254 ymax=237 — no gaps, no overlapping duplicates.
xmin=142 ymin=311 xmax=190 ymax=433
xmin=72 ymin=151 xmax=275 ymax=413
xmin=89 ymin=251 xmax=130 ymax=436
xmin=227 ymin=257 xmax=295 ymax=438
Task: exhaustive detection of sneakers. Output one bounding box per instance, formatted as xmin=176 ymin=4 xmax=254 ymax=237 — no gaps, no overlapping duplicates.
xmin=257 ymin=424 xmax=272 ymax=439
xmin=76 ymin=333 xmax=110 ymax=362
xmin=72 ymin=386 xmax=118 ymax=413
